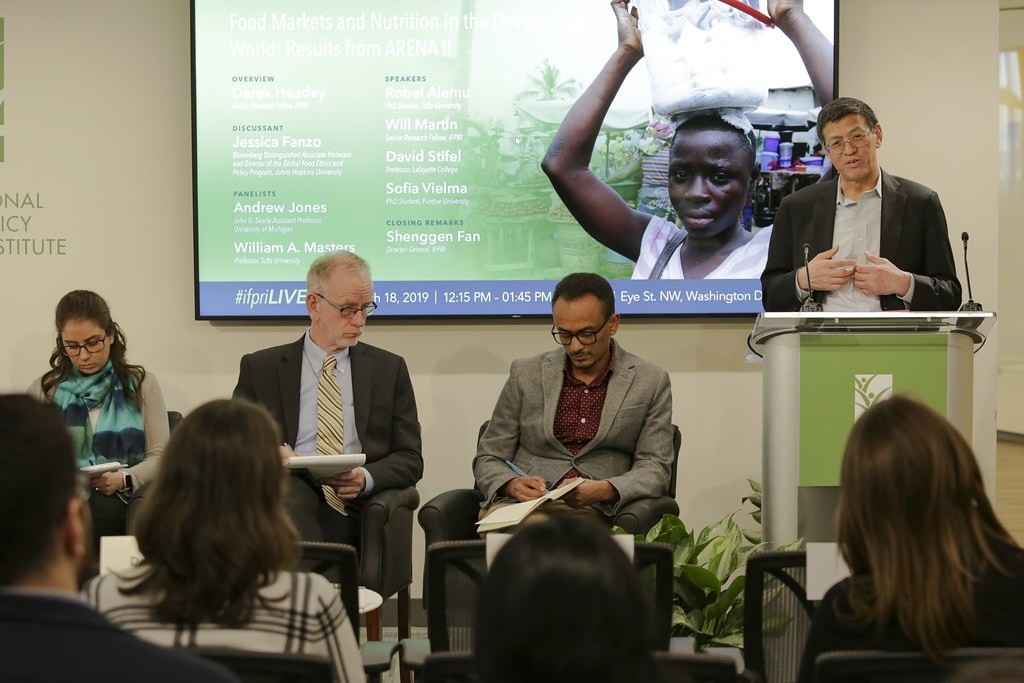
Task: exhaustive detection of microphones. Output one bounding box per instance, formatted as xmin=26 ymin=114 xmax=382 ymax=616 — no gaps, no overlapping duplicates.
xmin=799 ymin=243 xmax=822 ymax=312
xmin=955 ymin=232 xmax=985 ymax=329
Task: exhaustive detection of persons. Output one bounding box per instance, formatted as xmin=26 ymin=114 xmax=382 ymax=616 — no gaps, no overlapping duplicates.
xmin=26 ymin=290 xmax=171 ymax=552
xmin=470 ymin=515 xmax=659 ymax=683
xmin=541 ymin=0 xmax=834 ymax=280
xmin=232 ymin=252 xmax=425 ymax=647
xmin=760 ymin=97 xmax=962 ymax=312
xmin=0 ymin=393 xmax=240 ymax=683
xmin=472 ymin=272 xmax=674 ymax=540
xmin=77 ymin=400 xmax=366 ymax=683
xmin=796 ymin=393 xmax=1024 ymax=683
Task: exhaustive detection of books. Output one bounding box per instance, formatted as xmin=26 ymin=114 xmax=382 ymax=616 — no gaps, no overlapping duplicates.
xmin=284 ymin=454 xmax=366 ymax=481
xmin=79 ymin=462 xmax=128 ymax=475
xmin=477 ymin=477 xmax=585 ymax=533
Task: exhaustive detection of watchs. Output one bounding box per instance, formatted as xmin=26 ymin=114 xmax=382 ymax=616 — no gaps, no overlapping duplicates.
xmin=118 ymin=471 xmax=131 ymax=493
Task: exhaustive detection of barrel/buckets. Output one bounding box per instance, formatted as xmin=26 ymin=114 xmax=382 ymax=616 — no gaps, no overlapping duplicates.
xmin=760 ymin=130 xmax=807 ymax=172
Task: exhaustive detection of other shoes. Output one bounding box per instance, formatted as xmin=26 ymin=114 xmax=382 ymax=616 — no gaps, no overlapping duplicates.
xmin=330 ymin=582 xmax=383 ymax=614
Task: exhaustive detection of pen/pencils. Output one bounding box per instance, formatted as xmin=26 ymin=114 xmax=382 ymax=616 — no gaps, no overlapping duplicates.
xmin=505 ymin=458 xmax=550 ymax=494
xmin=89 ymin=459 xmax=94 ymax=466
xmin=284 ymin=442 xmax=294 ymax=453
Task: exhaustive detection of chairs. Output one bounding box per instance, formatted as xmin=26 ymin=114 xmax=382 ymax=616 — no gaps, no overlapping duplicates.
xmin=172 ymin=540 xmax=1024 ymax=683
xmin=353 ymin=486 xmax=418 ymax=646
xmin=418 ymin=420 xmax=683 ymax=654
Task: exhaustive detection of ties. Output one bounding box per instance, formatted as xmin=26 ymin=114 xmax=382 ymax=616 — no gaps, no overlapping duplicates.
xmin=316 ymin=355 xmax=349 ymax=516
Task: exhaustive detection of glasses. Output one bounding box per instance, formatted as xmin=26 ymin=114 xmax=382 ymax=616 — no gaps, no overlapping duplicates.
xmin=551 ymin=320 xmax=607 ymax=345
xmin=59 ymin=330 xmax=106 ymax=356
xmin=317 ymin=294 xmax=377 ymax=319
xmin=827 ymin=129 xmax=869 ymax=154
